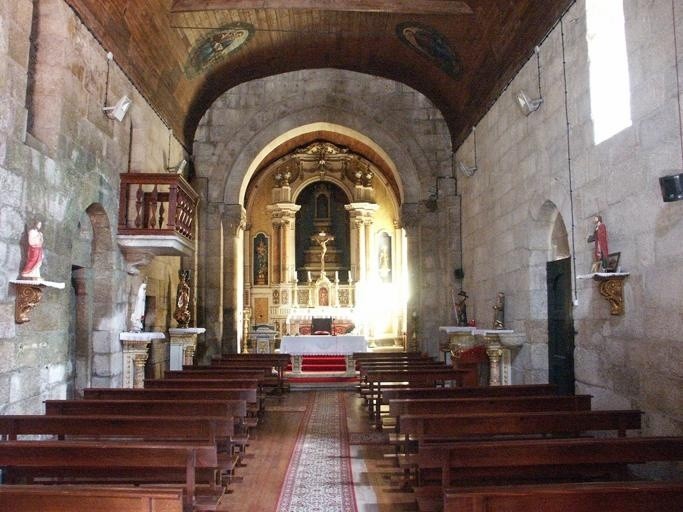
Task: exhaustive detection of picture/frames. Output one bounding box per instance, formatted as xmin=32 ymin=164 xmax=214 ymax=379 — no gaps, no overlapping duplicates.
xmin=607 ymin=252 xmax=620 ymax=272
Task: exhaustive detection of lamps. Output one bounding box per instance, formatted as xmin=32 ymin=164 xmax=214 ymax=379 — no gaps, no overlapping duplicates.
xmin=514 ymin=90 xmax=543 ymax=116
xmin=102 ymin=96 xmax=133 ymax=122
xmin=167 ymin=159 xmax=187 ymax=174
xmin=459 ymin=161 xmax=478 ymax=177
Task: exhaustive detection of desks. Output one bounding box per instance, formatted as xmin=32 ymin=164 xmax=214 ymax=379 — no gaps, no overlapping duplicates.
xmin=280 ymin=335 xmax=367 ymax=373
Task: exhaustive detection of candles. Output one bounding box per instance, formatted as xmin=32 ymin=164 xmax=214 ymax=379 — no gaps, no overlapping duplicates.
xmin=335 ymin=271 xmax=339 ymax=280
xmin=294 ymin=271 xmax=297 ymax=281
xmin=413 ymin=311 xmax=416 ymax=317
xmin=245 ymin=313 xmax=247 ymax=327
xmin=308 ymin=271 xmax=311 ymax=280
xmin=348 ymin=270 xmax=351 ymax=280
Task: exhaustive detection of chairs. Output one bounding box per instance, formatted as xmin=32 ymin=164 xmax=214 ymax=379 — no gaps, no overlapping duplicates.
xmin=310 ymin=316 xmax=332 ymax=335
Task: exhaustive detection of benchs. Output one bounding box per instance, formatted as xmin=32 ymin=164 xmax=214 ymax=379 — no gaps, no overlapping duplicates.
xmin=352 ymin=351 xmax=683 ymax=512
xmin=0 ymin=354 xmax=292 ymax=512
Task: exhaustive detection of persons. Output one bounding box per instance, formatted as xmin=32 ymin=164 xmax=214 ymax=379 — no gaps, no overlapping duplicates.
xmin=189 ymin=30 xmax=245 ymax=73
xmin=454 ymin=289 xmax=468 ymax=325
xmin=491 ymin=291 xmax=505 ymax=330
xmin=591 ymin=216 xmax=608 ymax=265
xmin=20 ymin=216 xmax=46 ymax=282
xmin=315 ymin=238 xmax=334 ymax=270
